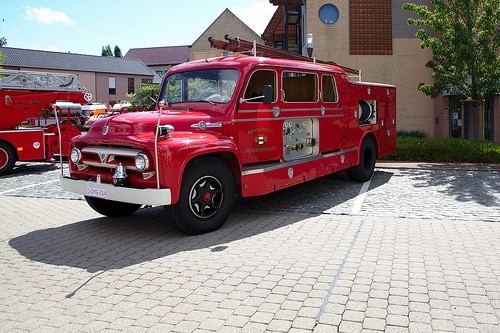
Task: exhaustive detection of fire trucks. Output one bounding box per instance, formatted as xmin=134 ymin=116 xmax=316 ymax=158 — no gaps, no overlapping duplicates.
xmin=0 ymin=69 xmax=92 ymax=176
xmin=81 ymin=100 xmax=150 ymax=132
xmin=50 ymin=32 xmax=398 ymax=236
